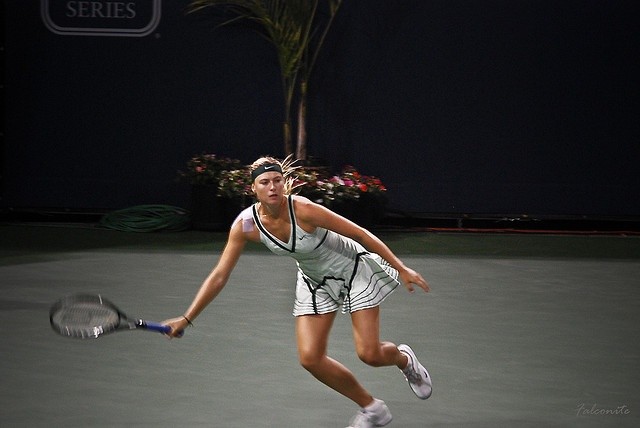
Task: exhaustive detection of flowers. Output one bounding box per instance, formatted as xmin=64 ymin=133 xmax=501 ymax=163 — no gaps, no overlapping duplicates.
xmin=303 ymin=164 xmax=348 ymax=204
xmin=187 ymin=154 xmax=237 ymax=184
xmin=344 ymin=167 xmax=386 ymax=198
xmin=220 ymin=165 xmax=259 ymax=198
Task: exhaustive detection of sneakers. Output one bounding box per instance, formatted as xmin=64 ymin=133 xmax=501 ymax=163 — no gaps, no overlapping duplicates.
xmin=345 ymin=400 xmax=392 ymax=428
xmin=396 ymin=343 xmax=432 ymax=400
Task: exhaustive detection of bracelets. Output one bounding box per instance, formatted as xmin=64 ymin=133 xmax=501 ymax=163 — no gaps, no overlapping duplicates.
xmin=182 ymin=314 xmax=191 ymax=325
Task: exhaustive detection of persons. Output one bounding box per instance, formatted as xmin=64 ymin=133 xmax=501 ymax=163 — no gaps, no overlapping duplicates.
xmin=160 ymin=152 xmax=432 ymax=428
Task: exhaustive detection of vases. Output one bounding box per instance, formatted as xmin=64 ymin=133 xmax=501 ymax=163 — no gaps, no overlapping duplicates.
xmin=225 ymin=196 xmax=252 ymax=231
xmin=188 ymin=176 xmax=238 ymax=231
xmin=354 ymin=200 xmax=376 ymax=231
xmin=320 ymin=201 xmax=350 ymax=219
